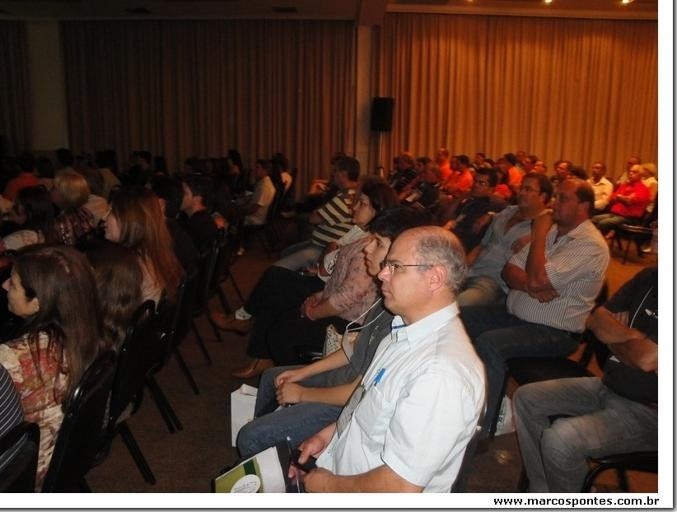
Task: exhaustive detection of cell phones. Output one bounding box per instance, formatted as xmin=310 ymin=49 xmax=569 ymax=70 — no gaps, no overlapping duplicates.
xmin=291 ymin=449 xmax=316 ymax=472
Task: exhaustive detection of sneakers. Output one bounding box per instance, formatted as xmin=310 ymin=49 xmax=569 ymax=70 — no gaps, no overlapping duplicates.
xmin=230 ymin=359 xmax=277 ymax=382
xmin=206 ymin=309 xmax=251 ymax=336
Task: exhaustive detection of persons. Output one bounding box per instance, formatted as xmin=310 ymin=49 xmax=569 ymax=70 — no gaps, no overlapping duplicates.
xmin=0 ymin=145 xmax=659 ymax=495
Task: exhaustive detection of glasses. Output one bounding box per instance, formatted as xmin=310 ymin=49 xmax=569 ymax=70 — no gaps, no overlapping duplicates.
xmin=380 ymin=260 xmax=429 ymax=273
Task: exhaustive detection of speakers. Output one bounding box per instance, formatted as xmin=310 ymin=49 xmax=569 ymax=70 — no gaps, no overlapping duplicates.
xmin=369 ymin=97 xmax=395 ymax=133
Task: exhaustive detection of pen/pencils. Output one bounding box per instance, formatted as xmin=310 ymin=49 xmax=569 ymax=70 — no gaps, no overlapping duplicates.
xmin=374 ymin=369 xmax=385 ymax=386
